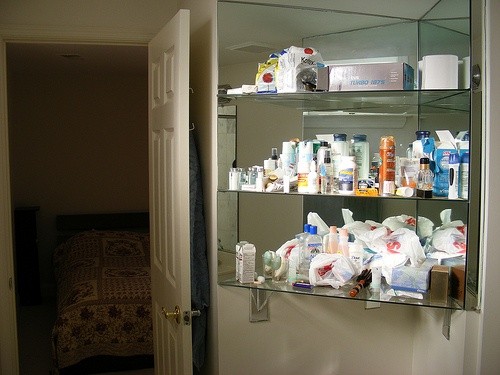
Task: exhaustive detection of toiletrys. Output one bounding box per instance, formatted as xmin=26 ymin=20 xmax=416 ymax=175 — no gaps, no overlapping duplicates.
xmin=295 ymin=223 xmax=322 ymax=278
xmin=229 ymin=133 xmax=369 ymax=195
xmin=449 ymin=152 xmax=469 ymax=200
xmin=322 ymin=225 xmax=349 ymax=257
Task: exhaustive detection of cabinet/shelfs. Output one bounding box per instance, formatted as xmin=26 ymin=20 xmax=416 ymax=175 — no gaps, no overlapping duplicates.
xmin=217 ymin=0 xmax=471 ymax=309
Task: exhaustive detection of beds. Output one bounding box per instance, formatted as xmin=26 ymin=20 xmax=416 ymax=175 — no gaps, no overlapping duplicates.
xmin=49 ymin=212 xmax=154 ymax=375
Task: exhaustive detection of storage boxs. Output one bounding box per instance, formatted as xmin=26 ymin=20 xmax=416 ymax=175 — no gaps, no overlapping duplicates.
xmin=328 ymin=62 xmax=415 ymax=92
xmin=454 ymin=130 xmax=470 ymax=155
xmin=236 ymin=241 xmax=249 ymax=280
xmin=434 ymin=130 xmax=458 ymax=195
xmin=239 ymin=244 xmax=256 ymax=284
xmin=431 ymin=265 xmax=452 ymax=305
xmin=450 ymin=266 xmax=466 ymax=299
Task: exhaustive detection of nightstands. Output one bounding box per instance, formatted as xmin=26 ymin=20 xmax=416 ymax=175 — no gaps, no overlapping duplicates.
xmin=14 ymin=207 xmax=42 ymax=305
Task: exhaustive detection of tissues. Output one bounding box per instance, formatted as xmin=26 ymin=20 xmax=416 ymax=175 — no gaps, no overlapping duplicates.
xmin=391 ymin=235 xmax=439 ymax=293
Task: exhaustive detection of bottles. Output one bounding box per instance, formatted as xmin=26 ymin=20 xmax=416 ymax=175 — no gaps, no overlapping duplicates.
xmin=228 ymin=130 xmax=470 ymax=201
xmin=296 ymin=223 xmax=349 ymax=276
